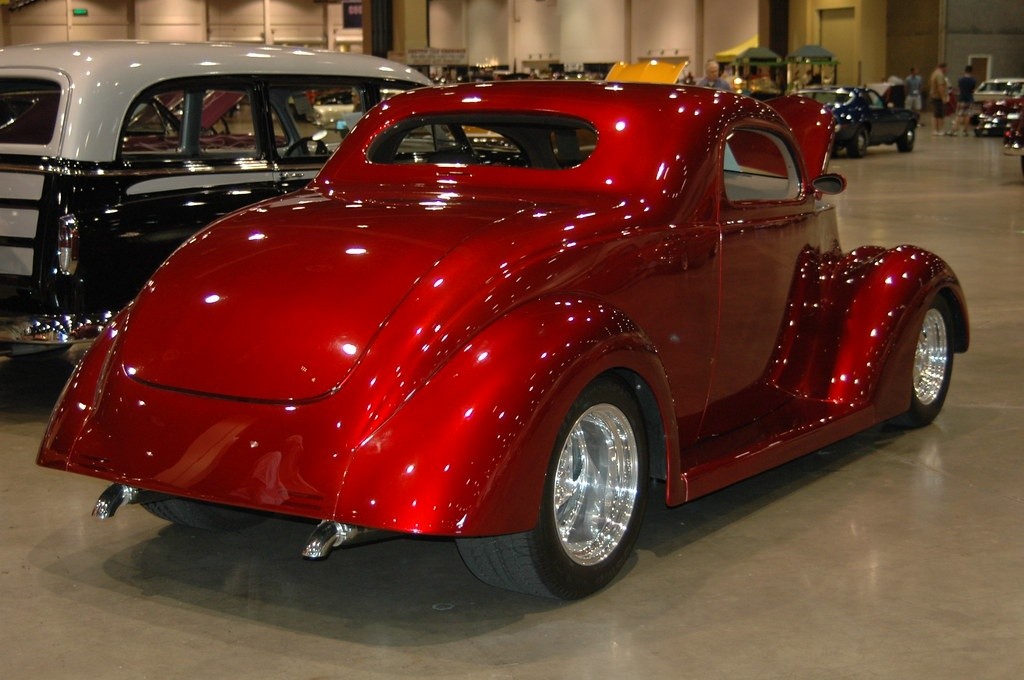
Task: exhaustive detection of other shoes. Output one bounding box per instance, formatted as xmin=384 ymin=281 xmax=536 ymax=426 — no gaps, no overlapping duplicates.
xmin=947 ymin=131 xmax=958 ymax=136
xmin=933 ymin=132 xmax=944 ymax=136
xmin=962 ymin=132 xmax=967 ymax=136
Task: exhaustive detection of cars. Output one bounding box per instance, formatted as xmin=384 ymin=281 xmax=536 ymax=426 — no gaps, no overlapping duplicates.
xmin=0 ymin=40 xmax=529 ymax=382
xmin=785 ymin=84 xmax=921 ymax=159
xmin=119 ymin=33 xmax=779 ymax=154
xmin=967 ymin=73 xmax=1024 ymax=178
xmin=36 ymin=76 xmax=975 ymax=604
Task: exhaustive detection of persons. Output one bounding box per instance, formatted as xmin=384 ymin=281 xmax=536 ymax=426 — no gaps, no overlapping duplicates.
xmin=946 ymin=66 xmax=976 ymax=137
xmin=930 ymin=63 xmax=950 ymax=135
xmin=698 ymin=59 xmax=730 ymax=91
xmin=907 ymin=68 xmax=923 ymax=126
xmin=807 ymin=71 xmax=822 ymax=86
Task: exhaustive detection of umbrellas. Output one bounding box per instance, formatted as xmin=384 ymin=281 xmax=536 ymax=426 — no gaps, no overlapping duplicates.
xmin=739 ymin=47 xmax=780 ymax=62
xmin=790 ymin=45 xmax=834 ymax=61
